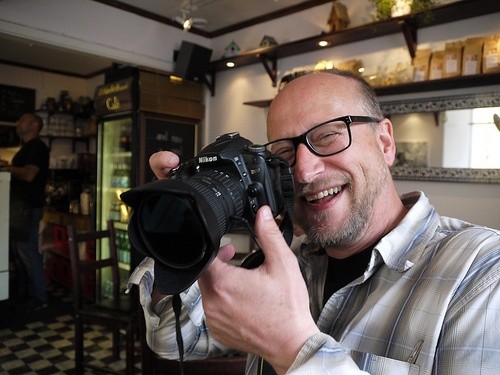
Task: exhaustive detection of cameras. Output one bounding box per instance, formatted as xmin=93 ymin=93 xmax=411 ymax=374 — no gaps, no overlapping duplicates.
xmin=120 ymin=131 xmax=283 ymax=295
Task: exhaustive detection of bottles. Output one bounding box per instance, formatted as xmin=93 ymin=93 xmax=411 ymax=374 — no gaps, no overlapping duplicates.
xmin=105 ymin=120 xmax=132 ymax=302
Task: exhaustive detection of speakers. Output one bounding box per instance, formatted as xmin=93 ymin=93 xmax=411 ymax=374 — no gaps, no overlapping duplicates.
xmin=173 ymin=41 xmax=213 ymax=86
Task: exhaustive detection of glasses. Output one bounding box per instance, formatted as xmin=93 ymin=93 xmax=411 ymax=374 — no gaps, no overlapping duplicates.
xmin=262 ymin=115 xmax=381 ymax=168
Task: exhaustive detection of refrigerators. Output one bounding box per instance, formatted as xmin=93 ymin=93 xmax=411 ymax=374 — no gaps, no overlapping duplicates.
xmin=91 ymin=108 xmax=204 ymax=328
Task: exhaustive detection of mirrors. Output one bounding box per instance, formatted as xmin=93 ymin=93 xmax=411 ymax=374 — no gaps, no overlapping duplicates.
xmin=379 ymin=91 xmax=500 ymax=185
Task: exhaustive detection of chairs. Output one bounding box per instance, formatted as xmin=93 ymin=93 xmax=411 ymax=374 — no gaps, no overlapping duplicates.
xmin=66 ymin=220 xmax=154 ymax=375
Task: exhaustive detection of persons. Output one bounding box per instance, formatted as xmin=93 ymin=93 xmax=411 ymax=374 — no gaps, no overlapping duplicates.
xmin=0 ymin=113 xmax=51 ymax=309
xmin=124 ymin=70 xmax=500 ymax=375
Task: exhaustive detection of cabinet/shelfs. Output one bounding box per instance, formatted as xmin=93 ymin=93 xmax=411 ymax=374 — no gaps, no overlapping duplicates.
xmin=203 ymin=0 xmax=500 ymax=108
